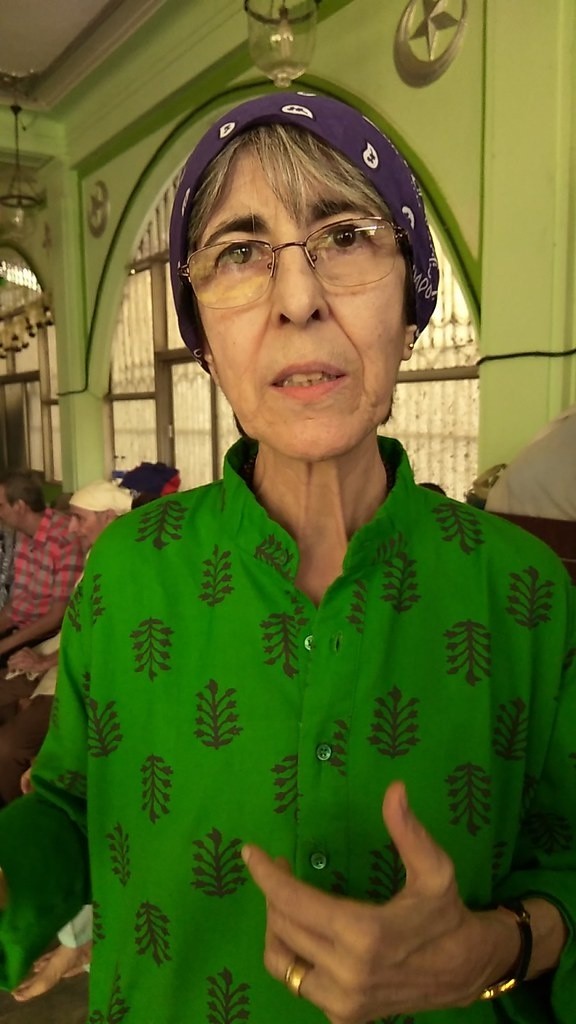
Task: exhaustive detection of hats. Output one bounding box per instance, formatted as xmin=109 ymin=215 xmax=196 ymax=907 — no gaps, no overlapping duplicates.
xmin=170 ymin=93 xmax=439 ymax=376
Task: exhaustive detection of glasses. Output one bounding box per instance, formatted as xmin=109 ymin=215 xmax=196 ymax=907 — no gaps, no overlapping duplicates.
xmin=178 ymin=217 xmax=410 ymax=289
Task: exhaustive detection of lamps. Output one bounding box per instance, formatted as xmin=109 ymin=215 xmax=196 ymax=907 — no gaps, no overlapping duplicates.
xmin=1 ymin=102 xmax=45 ymax=240
xmin=245 ymin=0 xmax=318 ymax=88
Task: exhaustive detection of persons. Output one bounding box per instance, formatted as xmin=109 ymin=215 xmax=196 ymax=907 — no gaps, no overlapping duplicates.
xmin=0 ymin=473 xmax=87 ymax=670
xmin=1 ymin=478 xmax=140 ymax=810
xmin=0 ymin=90 xmax=574 ymax=1023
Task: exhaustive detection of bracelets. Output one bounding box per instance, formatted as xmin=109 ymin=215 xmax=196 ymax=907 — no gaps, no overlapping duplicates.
xmin=480 ymin=898 xmax=533 ymax=1000
